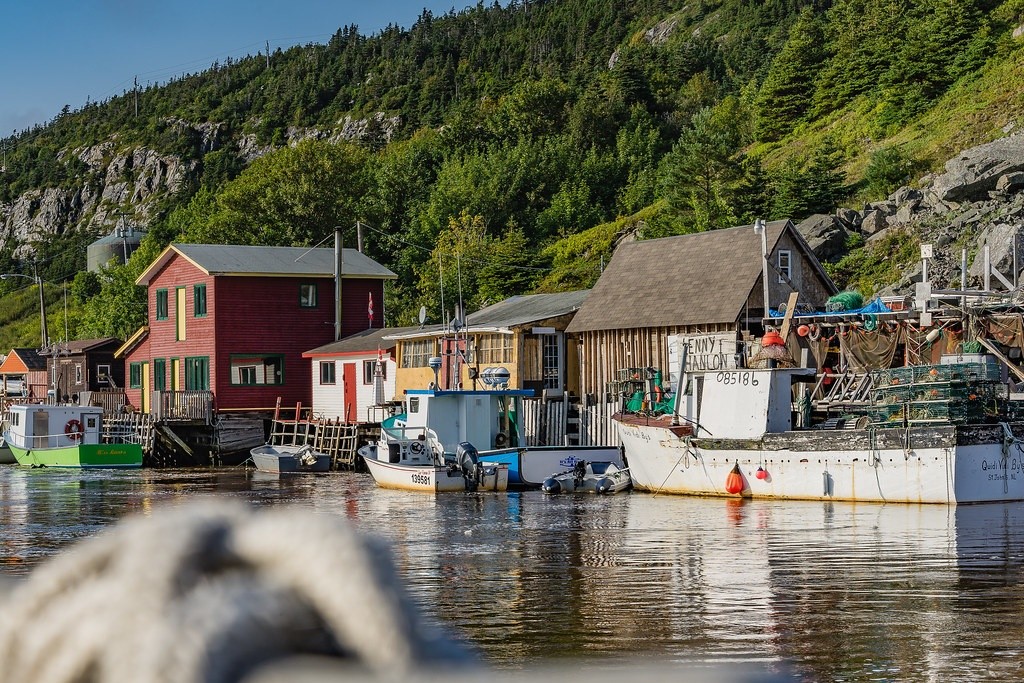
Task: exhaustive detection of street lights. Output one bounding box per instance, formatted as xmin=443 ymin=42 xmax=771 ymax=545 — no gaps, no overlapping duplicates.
xmin=0 ymin=274 xmax=48 ymax=348
xmin=754 ymin=217 xmax=773 ymax=369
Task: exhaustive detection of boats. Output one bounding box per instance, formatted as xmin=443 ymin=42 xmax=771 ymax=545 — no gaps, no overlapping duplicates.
xmin=381 ymin=317 xmax=623 ymax=490
xmin=612 ymin=333 xmax=1024 ymax=504
xmin=250 ymin=444 xmax=331 ymax=472
xmin=0 ymin=437 xmax=18 ymax=463
xmin=4 ymin=373 xmax=143 ymax=467
xmin=357 ymin=426 xmax=512 ymax=493
xmin=541 ymin=461 xmax=632 ymax=496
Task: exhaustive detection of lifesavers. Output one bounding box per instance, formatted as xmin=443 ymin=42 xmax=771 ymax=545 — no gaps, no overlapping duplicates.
xmin=644 ymin=386 xmax=662 ymax=402
xmin=495 ymin=433 xmax=506 ymax=446
xmin=65 ymin=420 xmax=84 ymax=440
